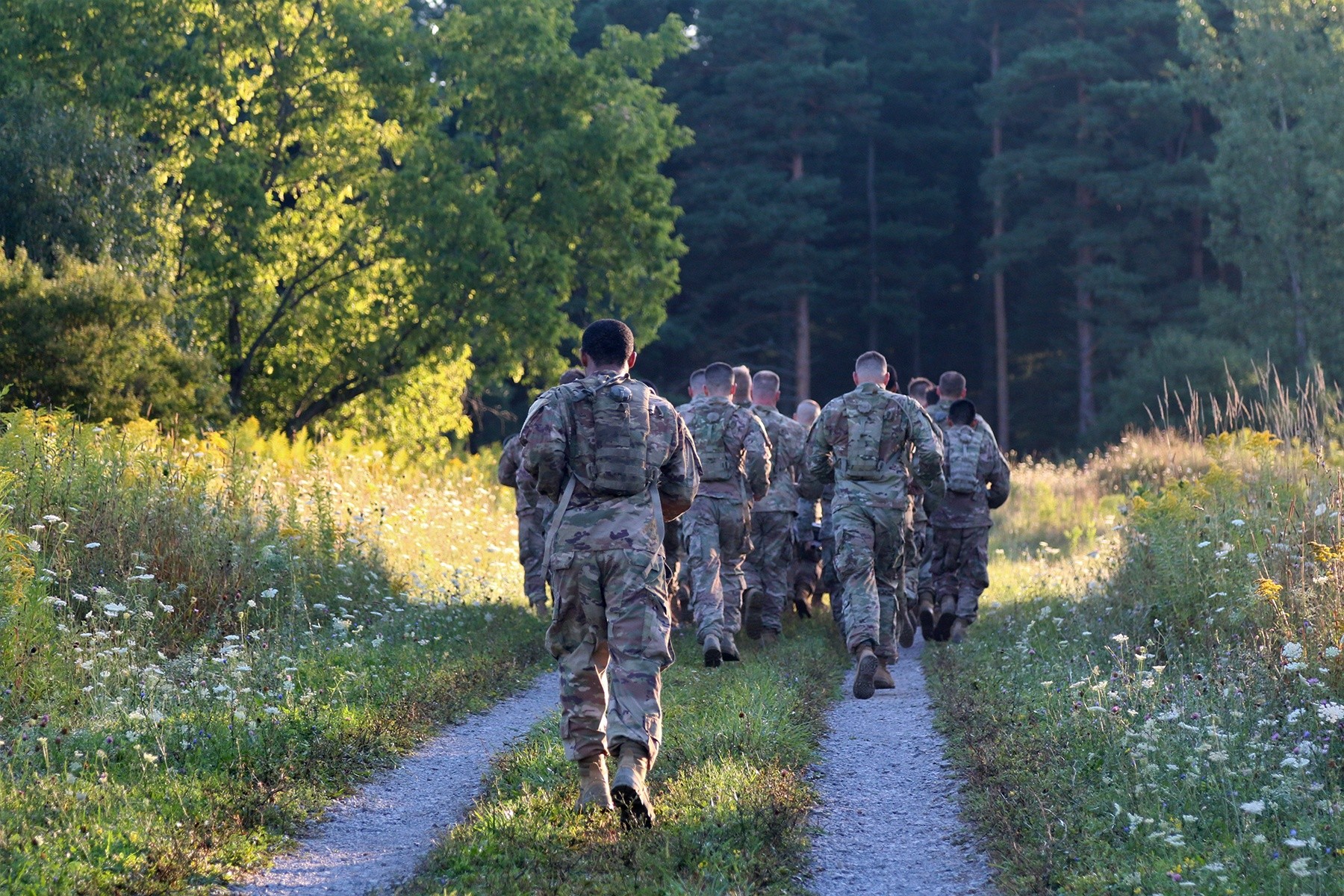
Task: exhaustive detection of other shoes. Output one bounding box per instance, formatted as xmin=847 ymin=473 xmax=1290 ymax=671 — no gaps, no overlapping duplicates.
xmin=888 ymin=600 xmax=936 ymax=664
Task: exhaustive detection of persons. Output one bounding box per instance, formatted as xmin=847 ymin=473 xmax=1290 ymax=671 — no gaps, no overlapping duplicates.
xmin=732 ymin=366 xmax=752 ymax=408
xmin=683 ymin=361 xmax=773 ymax=668
xmin=519 ymin=317 xmax=705 ymax=836
xmin=885 ymin=362 xmax=997 ymax=648
xmin=787 ymin=397 xmax=846 ymax=644
xmin=804 ymin=351 xmax=945 ymax=700
xmin=673 ymin=368 xmax=708 ymax=624
xmin=743 ymin=371 xmax=807 ymax=647
xmin=638 ymin=378 xmax=684 ymax=624
xmin=923 ymin=398 xmax=1011 ymax=644
xmin=497 ymin=370 xmax=587 ymax=620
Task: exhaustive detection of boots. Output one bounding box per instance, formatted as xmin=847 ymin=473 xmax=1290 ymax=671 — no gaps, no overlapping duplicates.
xmin=744 ymin=590 xmax=778 ymax=647
xmin=934 ymin=595 xmax=969 ymax=643
xmin=668 ymin=593 xmax=680 ymax=628
xmin=785 ymin=583 xmax=813 ymax=619
xmin=609 ymin=740 xmax=656 ymax=832
xmin=536 ymin=598 xmax=548 ymax=618
xmin=677 ymin=579 xmax=695 ymax=614
xmin=703 ymin=631 xmax=741 ymax=666
xmin=810 ymin=592 xmax=828 ymax=609
xmin=573 ymin=753 xmax=615 ymax=813
xmin=851 ymin=644 xmax=896 ymax=699
